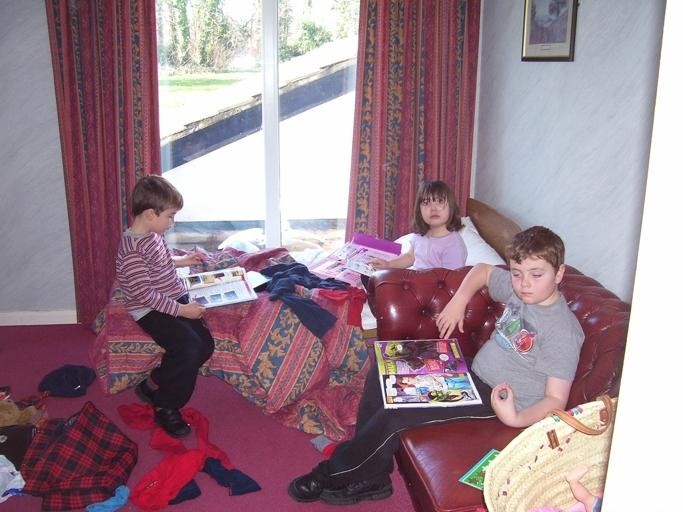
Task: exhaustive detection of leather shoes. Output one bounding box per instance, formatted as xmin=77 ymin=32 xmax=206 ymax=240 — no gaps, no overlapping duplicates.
xmin=287 ymin=460 xmax=393 ymax=505
xmin=133 ymin=377 xmax=191 ymax=438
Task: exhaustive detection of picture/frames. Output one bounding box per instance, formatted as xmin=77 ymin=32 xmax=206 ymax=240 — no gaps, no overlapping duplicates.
xmin=521 ymin=0 xmax=578 ymax=62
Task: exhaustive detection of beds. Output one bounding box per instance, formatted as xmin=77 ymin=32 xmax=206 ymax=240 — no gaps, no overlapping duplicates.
xmin=93 ymin=198 xmax=522 ymax=396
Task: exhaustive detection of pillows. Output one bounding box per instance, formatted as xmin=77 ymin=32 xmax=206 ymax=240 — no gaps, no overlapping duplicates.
xmin=458 ymin=217 xmax=506 ymax=266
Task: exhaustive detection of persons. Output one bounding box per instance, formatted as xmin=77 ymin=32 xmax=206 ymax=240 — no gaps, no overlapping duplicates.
xmin=565 ymin=463 xmax=602 ymax=512
xmin=114 ymin=175 xmax=214 ymax=438
xmin=366 ymin=179 xmax=467 ymax=269
xmin=286 ymin=225 xmax=588 ymax=505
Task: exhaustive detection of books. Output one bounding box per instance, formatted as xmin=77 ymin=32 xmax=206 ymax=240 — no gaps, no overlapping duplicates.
xmin=372 ymin=337 xmax=483 ymax=409
xmin=181 ymin=265 xmax=258 ymax=308
xmin=310 ymin=240 xmax=399 ymax=291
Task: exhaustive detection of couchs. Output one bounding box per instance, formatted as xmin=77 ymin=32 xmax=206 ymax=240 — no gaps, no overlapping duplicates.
xmin=361 ymin=263 xmax=632 ymax=512
